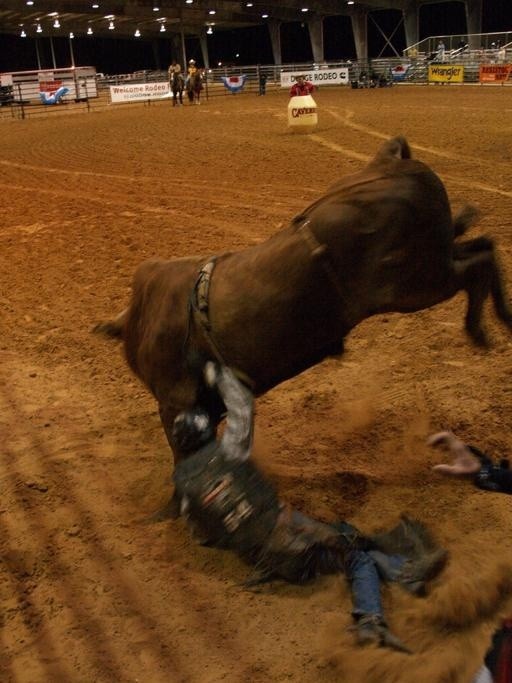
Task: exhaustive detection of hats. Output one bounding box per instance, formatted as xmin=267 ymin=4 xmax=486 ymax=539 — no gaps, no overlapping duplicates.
xmin=291 ymin=72 xmax=308 ymax=78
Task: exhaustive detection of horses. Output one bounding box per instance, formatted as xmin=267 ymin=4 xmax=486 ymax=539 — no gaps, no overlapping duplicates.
xmin=170 ymin=69 xmax=184 ymax=107
xmin=186 ymin=68 xmax=201 ymax=105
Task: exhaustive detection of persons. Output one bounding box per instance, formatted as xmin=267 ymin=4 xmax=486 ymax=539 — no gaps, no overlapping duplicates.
xmin=290 ymin=76 xmax=314 ymax=97
xmin=184 ymin=58 xmax=198 ymax=86
xmin=437 ymin=38 xmax=445 ymax=62
xmin=426 ymin=431 xmax=512 ymax=494
xmin=407 ymin=46 xmax=418 ymax=66
xmin=479 ymin=46 xmax=505 ymax=65
xmin=359 ymin=72 xmax=368 ymax=81
xmin=260 ymin=71 xmax=267 ymax=96
xmin=171 ymin=361 xmax=451 ymax=654
xmin=168 ymin=58 xmax=184 ymax=80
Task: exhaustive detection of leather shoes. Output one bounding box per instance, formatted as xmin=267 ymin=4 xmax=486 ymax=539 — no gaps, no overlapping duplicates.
xmin=409 ymin=550 xmax=450 ymax=598
xmin=357 ymin=624 xmax=414 ymax=655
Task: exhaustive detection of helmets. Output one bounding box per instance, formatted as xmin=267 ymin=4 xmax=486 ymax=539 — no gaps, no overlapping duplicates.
xmin=169 ymin=409 xmax=215 ymax=452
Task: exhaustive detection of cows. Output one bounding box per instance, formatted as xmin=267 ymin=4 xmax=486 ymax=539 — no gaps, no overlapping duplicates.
xmin=91 ymin=136 xmax=512 ymax=520
